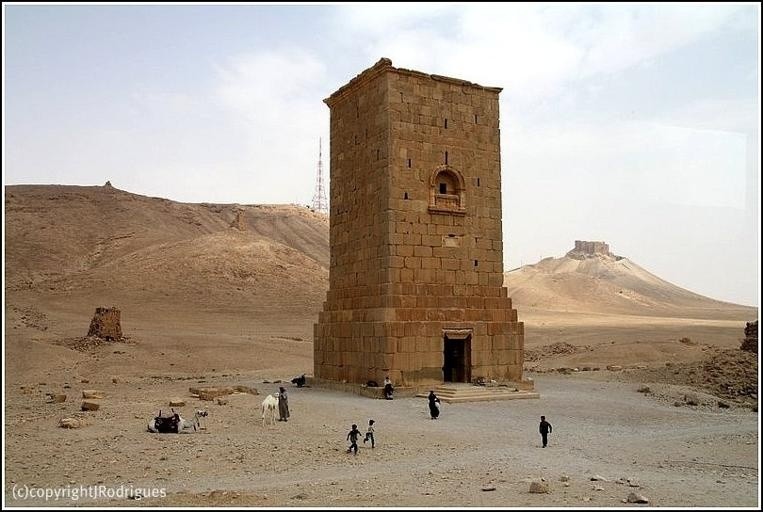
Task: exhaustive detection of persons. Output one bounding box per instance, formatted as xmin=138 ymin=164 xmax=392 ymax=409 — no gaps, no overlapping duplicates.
xmin=539 ymin=416 xmax=552 ymax=448
xmin=346 ymin=425 xmax=361 ymax=455
xmin=428 ymin=390 xmax=440 ymax=420
xmin=383 ymin=376 xmax=393 ymax=399
xmin=278 ymin=387 xmax=289 ymax=422
xmin=363 ymin=420 xmax=376 ymax=448
